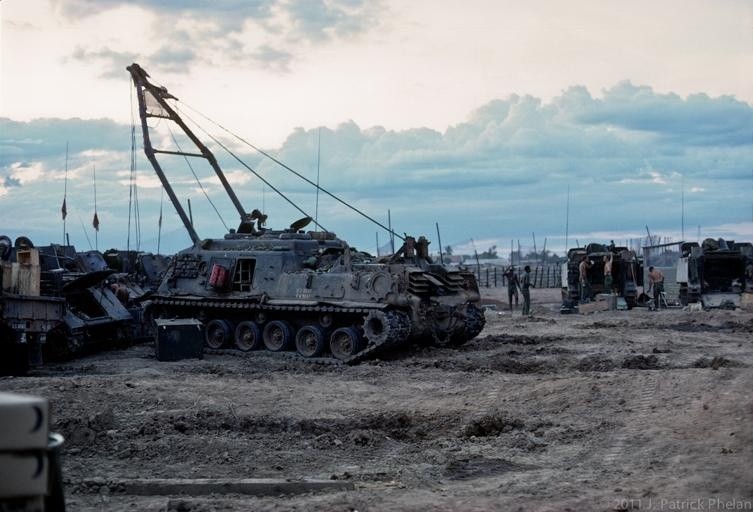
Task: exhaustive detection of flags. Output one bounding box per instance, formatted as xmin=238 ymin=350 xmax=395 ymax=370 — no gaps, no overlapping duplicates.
xmin=91 ymin=214 xmax=99 ymax=231
xmin=60 ymin=199 xmax=67 ymax=220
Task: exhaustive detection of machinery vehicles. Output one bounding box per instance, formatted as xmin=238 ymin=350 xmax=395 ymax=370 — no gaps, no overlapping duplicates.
xmin=675 ymin=239 xmax=753 ymax=310
xmin=114 ymin=60 xmax=487 ymax=366
xmin=557 ymin=242 xmax=646 ymax=311
xmin=0 ymin=233 xmax=171 ymax=379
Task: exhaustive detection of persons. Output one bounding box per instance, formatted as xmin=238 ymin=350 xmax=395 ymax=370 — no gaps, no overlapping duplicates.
xmin=578 ymin=254 xmax=595 ymax=304
xmin=645 ymin=265 xmax=665 ymax=309
xmin=502 ymin=267 xmax=521 ymax=312
xmin=520 ymin=265 xmax=535 ymax=316
xmin=602 ymin=250 xmax=614 ymax=294
